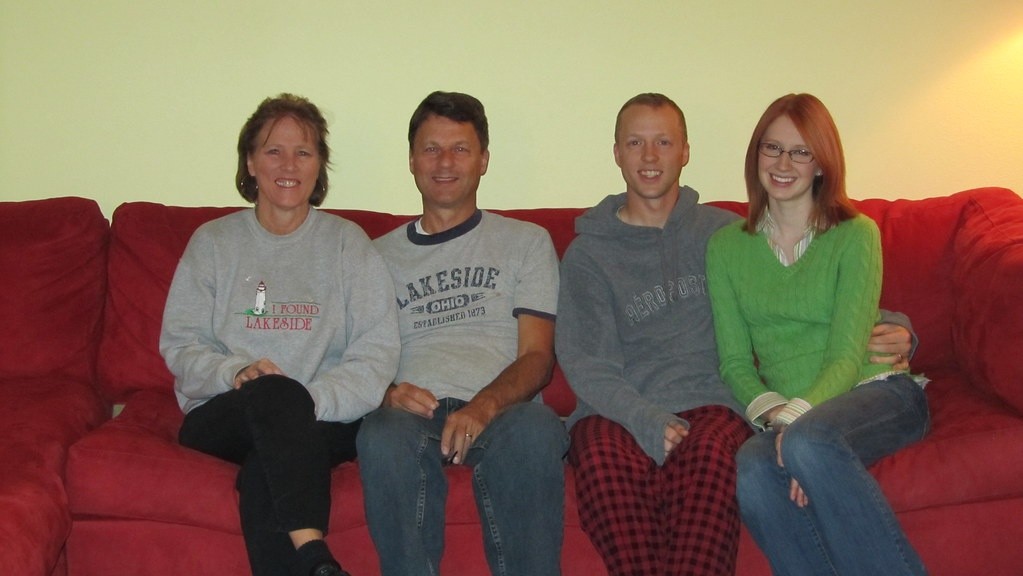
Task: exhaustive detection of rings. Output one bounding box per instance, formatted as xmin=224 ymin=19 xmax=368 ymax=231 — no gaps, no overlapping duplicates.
xmin=898 ymin=353 xmax=903 ymax=360
xmin=465 ymin=433 xmax=472 ymax=437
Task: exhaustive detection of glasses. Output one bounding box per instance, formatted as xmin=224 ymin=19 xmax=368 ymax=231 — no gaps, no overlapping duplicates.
xmin=758 ymin=142 xmax=814 ymax=164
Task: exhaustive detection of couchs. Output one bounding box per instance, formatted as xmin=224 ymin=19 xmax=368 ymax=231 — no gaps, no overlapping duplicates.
xmin=0 ymin=195 xmax=115 ymax=576
xmin=64 ymin=185 xmax=1023 ymax=576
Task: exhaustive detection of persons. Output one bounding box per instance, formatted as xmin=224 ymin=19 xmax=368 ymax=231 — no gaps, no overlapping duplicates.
xmin=354 ymin=92 xmax=573 ymax=576
xmin=555 ymin=92 xmax=922 ymax=576
xmin=705 ymin=93 xmax=937 ymax=576
xmin=156 ymin=91 xmax=399 ymax=575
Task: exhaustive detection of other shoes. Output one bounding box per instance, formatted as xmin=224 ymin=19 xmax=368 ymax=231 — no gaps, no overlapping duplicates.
xmin=311 ymin=562 xmax=344 ymax=576
